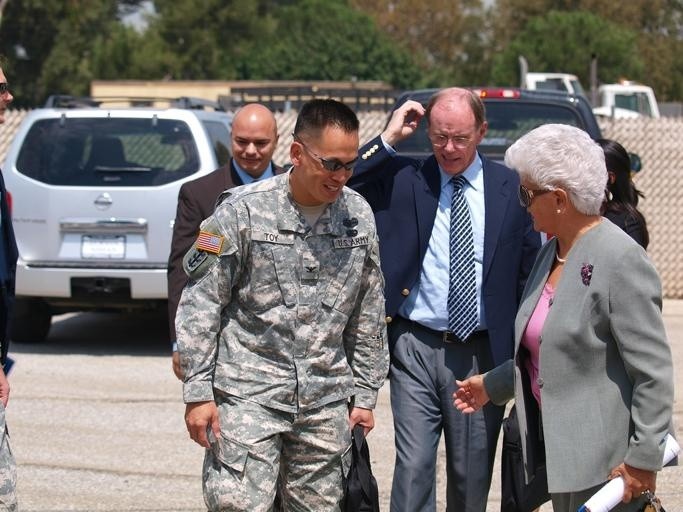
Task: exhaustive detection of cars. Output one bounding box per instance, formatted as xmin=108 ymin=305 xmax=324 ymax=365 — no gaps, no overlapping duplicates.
xmin=382 ymin=71 xmax=666 ymax=166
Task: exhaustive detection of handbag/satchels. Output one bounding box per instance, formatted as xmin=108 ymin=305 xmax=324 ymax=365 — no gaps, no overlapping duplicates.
xmin=500 ymin=402 xmax=551 ymax=512
xmin=339 ymin=425 xmax=380 ymax=512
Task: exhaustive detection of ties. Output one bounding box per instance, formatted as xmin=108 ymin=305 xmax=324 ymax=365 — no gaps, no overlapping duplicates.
xmin=446 ymin=175 xmax=480 ymax=342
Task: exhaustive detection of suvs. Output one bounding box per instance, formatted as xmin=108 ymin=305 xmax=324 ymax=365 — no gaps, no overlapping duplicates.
xmin=0 ymin=95 xmax=234 ymax=345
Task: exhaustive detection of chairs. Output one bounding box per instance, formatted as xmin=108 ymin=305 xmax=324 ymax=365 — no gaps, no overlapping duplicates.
xmin=86 ymin=138 xmax=126 ymax=168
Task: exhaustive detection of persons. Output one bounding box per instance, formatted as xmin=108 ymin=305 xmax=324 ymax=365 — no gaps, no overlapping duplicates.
xmin=0 ymin=67 xmax=21 ymax=511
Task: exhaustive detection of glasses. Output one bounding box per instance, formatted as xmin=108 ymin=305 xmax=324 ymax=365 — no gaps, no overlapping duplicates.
xmin=0 ymin=83 xmax=8 ymax=94
xmin=428 ymin=134 xmax=472 ymax=147
xmin=290 ymin=132 xmax=359 ymax=171
xmin=518 ymin=184 xmax=553 ymax=207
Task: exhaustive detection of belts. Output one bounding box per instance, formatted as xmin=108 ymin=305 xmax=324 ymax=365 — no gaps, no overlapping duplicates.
xmin=404 ymin=316 xmax=488 ymax=344
